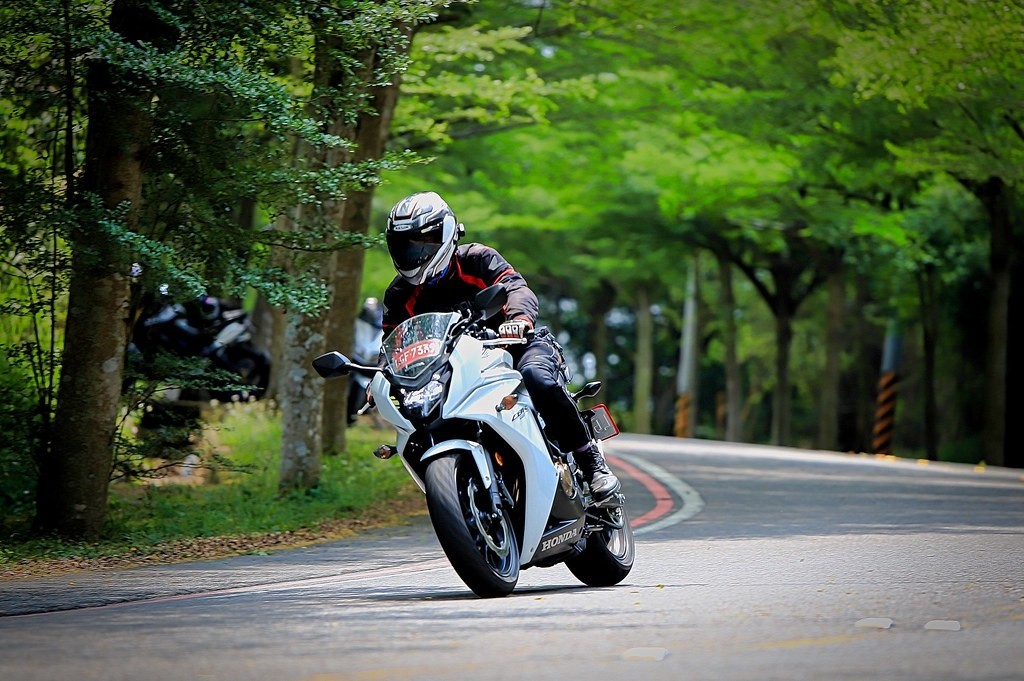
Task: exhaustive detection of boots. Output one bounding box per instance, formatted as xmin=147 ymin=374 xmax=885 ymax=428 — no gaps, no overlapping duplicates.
xmin=572 ymin=438 xmax=622 ymax=502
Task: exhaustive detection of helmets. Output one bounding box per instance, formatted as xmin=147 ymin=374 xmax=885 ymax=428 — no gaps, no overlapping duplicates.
xmin=385 ymin=190 xmax=466 ymax=291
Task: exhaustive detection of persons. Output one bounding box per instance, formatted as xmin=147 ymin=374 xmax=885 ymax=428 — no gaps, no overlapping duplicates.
xmin=361 ymin=297 xmax=383 ymax=328
xmin=366 ymin=190 xmax=622 ymax=502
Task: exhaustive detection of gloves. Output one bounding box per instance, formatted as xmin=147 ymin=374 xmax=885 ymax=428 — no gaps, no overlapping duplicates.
xmin=497 ymin=314 xmax=535 ymax=349
xmin=364 ymin=378 xmax=375 ymax=408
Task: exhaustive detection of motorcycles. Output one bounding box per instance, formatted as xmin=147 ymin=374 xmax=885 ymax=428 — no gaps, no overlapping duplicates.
xmin=122 ymin=263 xmax=273 ymax=400
xmin=347 ymin=307 xmax=383 ymax=427
xmin=312 ymin=283 xmax=635 ymax=596
